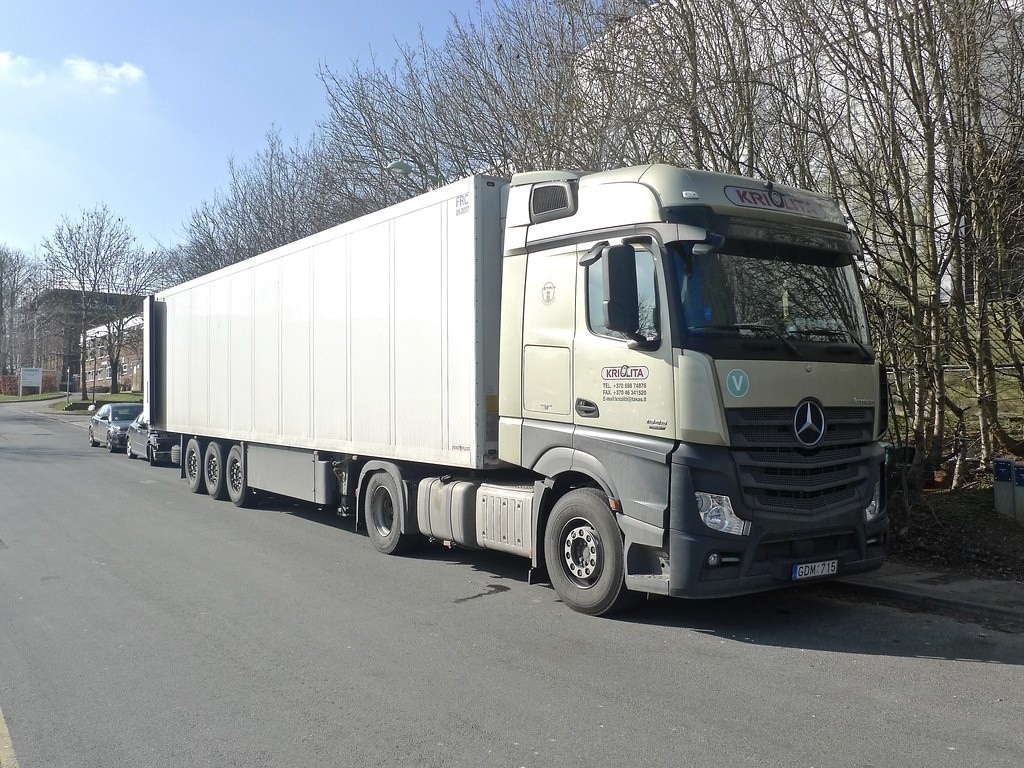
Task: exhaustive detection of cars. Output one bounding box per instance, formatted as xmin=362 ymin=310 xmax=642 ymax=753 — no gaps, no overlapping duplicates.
xmin=878 ymin=443 xmax=935 ymax=490
xmin=124 ymin=410 xmax=181 ymax=467
xmin=88 ymin=403 xmax=144 ymax=453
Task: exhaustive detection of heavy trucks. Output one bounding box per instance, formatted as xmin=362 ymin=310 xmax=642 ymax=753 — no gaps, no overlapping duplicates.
xmin=140 ymin=160 xmax=897 ymax=619
